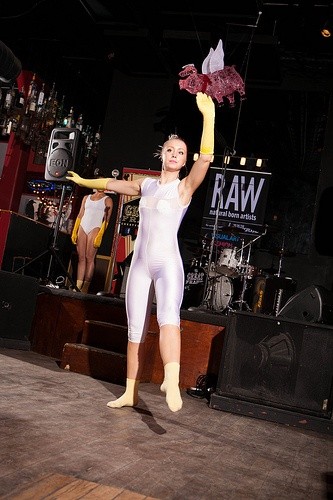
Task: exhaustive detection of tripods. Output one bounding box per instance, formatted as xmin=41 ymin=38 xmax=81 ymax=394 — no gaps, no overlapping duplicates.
xmin=14 ymin=185 xmax=81 ymax=293
xmin=220 ymin=228 xmax=269 ymax=312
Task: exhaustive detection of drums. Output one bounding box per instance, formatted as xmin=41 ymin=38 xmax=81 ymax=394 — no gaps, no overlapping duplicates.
xmin=183 ymin=265 xmax=233 ymax=313
xmin=217 ymin=249 xmax=243 ymax=277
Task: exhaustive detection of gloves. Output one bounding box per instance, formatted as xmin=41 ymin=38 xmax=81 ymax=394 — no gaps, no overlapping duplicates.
xmin=196 ymin=91 xmax=215 ymax=156
xmin=71 ymin=218 xmax=80 ymax=245
xmin=93 ymin=221 xmax=108 ymax=248
xmin=66 ymin=171 xmax=110 ymax=191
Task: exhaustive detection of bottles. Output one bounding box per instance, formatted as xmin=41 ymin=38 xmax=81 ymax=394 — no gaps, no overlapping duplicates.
xmin=0 ymin=73 xmax=103 ymax=167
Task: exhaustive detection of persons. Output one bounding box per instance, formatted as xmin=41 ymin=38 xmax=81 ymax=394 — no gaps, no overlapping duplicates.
xmin=65 ymin=91 xmax=215 ymax=413
xmin=71 ymin=177 xmax=113 ymax=294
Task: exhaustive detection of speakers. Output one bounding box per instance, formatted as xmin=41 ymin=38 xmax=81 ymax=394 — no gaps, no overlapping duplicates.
xmin=45 ymin=128 xmax=82 ymax=185
xmin=65 ymin=251 xmax=111 ymax=294
xmin=215 ymin=284 xmax=333 ymax=419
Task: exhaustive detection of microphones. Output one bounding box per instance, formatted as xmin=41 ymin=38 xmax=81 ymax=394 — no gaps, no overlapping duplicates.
xmin=226 ymin=146 xmax=236 ymax=155
